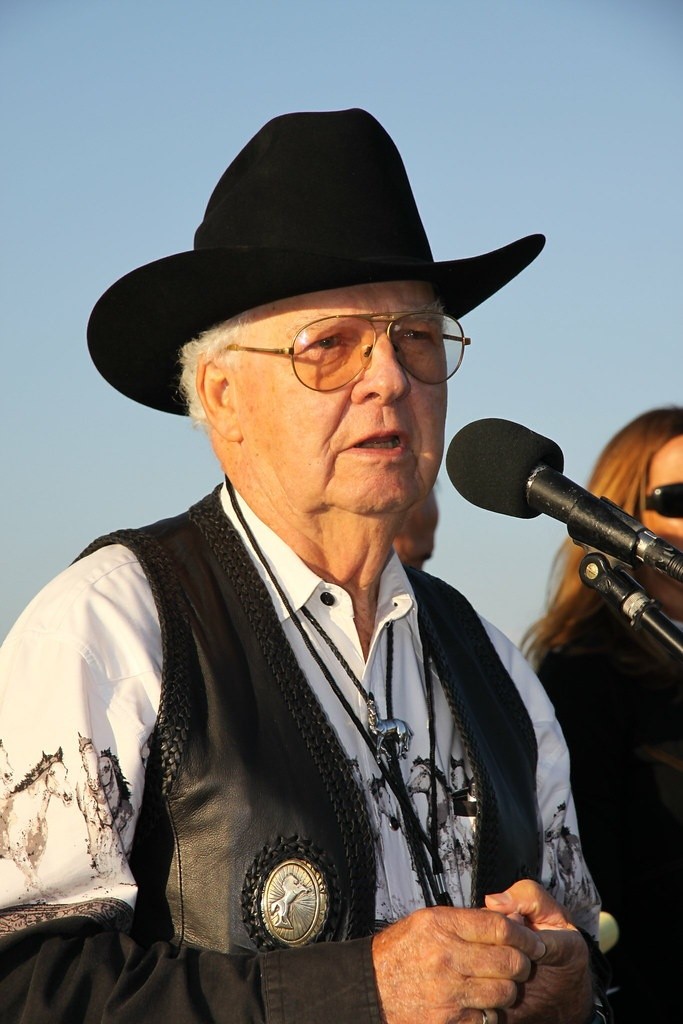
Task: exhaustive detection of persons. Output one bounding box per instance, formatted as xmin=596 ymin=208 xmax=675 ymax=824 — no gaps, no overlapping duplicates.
xmin=525 ymin=406 xmax=683 ymax=1024
xmin=392 ymin=488 xmax=439 ymax=569
xmin=0 ymin=108 xmax=621 ymax=1024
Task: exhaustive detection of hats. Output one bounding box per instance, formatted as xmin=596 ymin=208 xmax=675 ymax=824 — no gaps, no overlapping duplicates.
xmin=86 ymin=107 xmax=546 ymax=417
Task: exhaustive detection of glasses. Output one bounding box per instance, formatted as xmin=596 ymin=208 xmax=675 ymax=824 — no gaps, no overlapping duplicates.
xmin=225 ymin=311 xmax=472 ymax=392
xmin=646 ymin=482 xmax=683 ymax=518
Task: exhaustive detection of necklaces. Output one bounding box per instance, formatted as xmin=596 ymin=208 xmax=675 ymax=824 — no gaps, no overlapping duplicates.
xmin=225 ymin=475 xmax=454 ymax=907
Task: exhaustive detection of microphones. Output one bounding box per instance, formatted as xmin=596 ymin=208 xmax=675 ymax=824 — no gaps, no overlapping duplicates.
xmin=446 ymin=418 xmax=683 ymax=583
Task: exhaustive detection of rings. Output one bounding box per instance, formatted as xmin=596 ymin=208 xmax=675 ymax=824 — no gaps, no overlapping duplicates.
xmin=481 ymin=1009 xmax=487 ymax=1024
